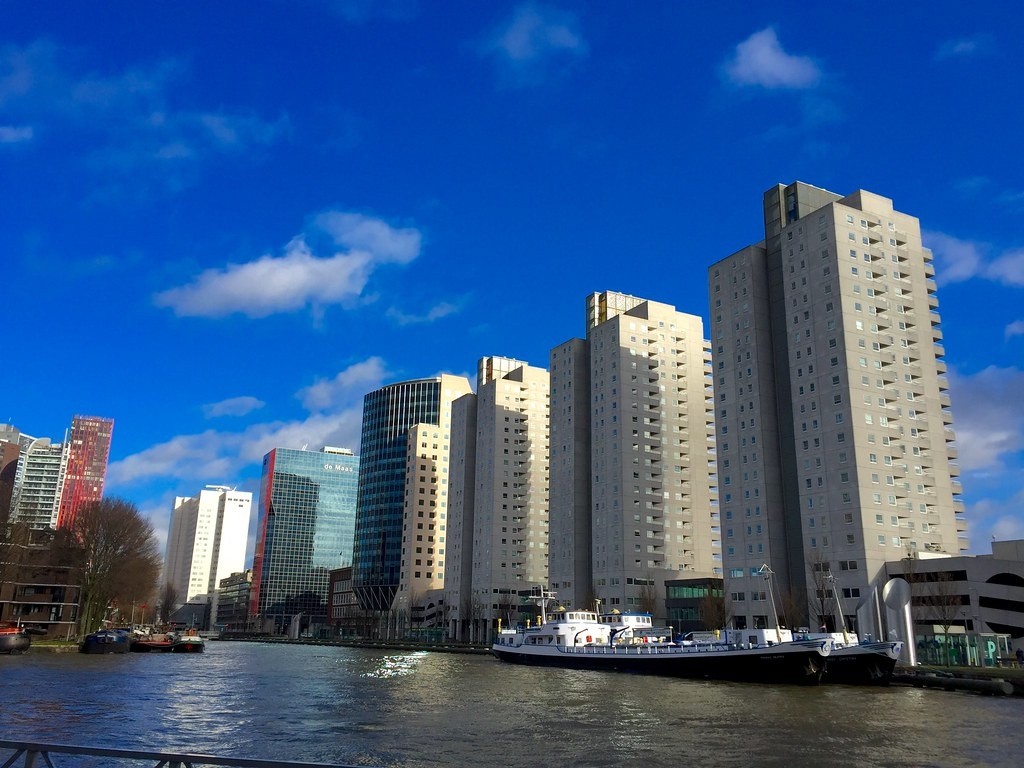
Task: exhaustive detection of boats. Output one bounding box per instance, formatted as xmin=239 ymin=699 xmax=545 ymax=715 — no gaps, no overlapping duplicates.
xmin=78 ymin=626 xmax=132 ymax=655
xmin=169 ymin=624 xmax=206 ymax=654
xmin=489 ymin=563 xmax=906 ymax=688
xmin=130 ymin=622 xmax=176 ymax=654
xmin=0 ymin=616 xmax=31 ymax=655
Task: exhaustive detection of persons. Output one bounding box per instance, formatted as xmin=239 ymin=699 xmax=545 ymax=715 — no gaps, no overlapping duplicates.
xmin=714 ymin=627 xmax=720 ymax=641
xmin=1016 ymin=648 xmax=1024 ymax=668
xmin=656 ymin=636 xmax=663 ymax=644
